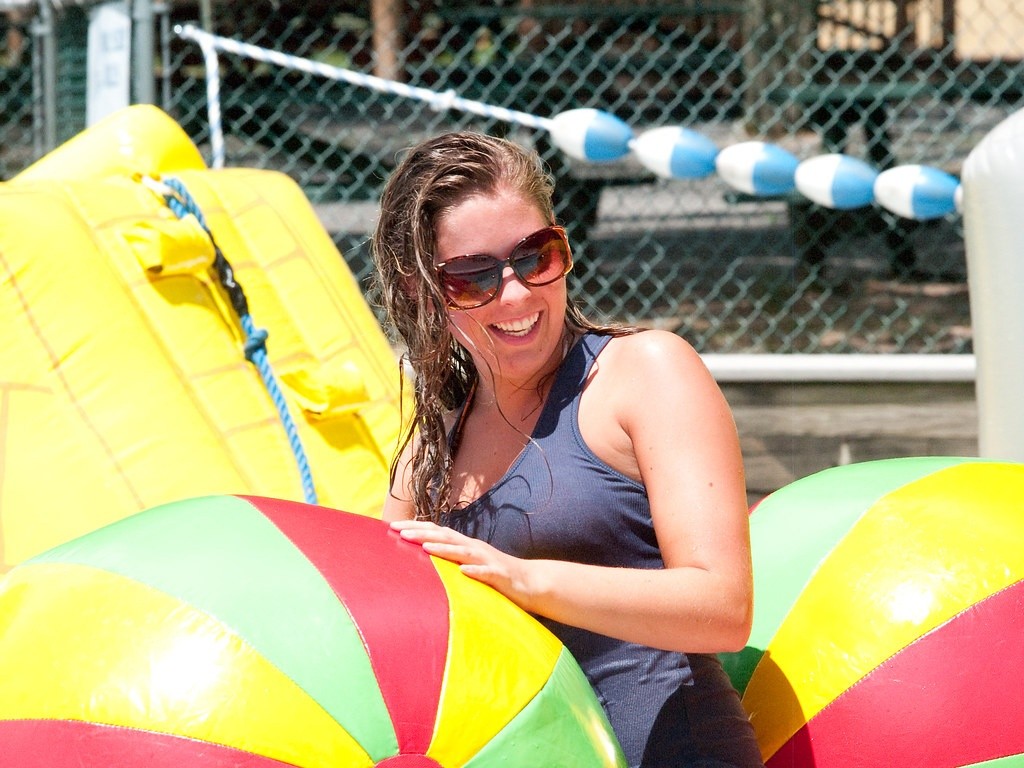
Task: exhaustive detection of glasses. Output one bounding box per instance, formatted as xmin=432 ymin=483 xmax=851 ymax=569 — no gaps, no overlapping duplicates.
xmin=429 ymin=226 xmax=574 ymax=311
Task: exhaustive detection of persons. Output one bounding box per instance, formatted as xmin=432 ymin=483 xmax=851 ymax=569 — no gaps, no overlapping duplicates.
xmin=377 ymin=132 xmax=766 ymax=768
xmin=787 ymin=51 xmax=923 ymax=298
xmin=482 ymin=0 xmax=621 ymax=287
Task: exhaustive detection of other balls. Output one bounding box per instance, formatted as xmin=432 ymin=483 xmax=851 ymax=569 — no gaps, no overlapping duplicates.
xmin=710 ymin=453 xmax=1024 ymax=767
xmin=0 ymin=491 xmax=630 ymax=767
